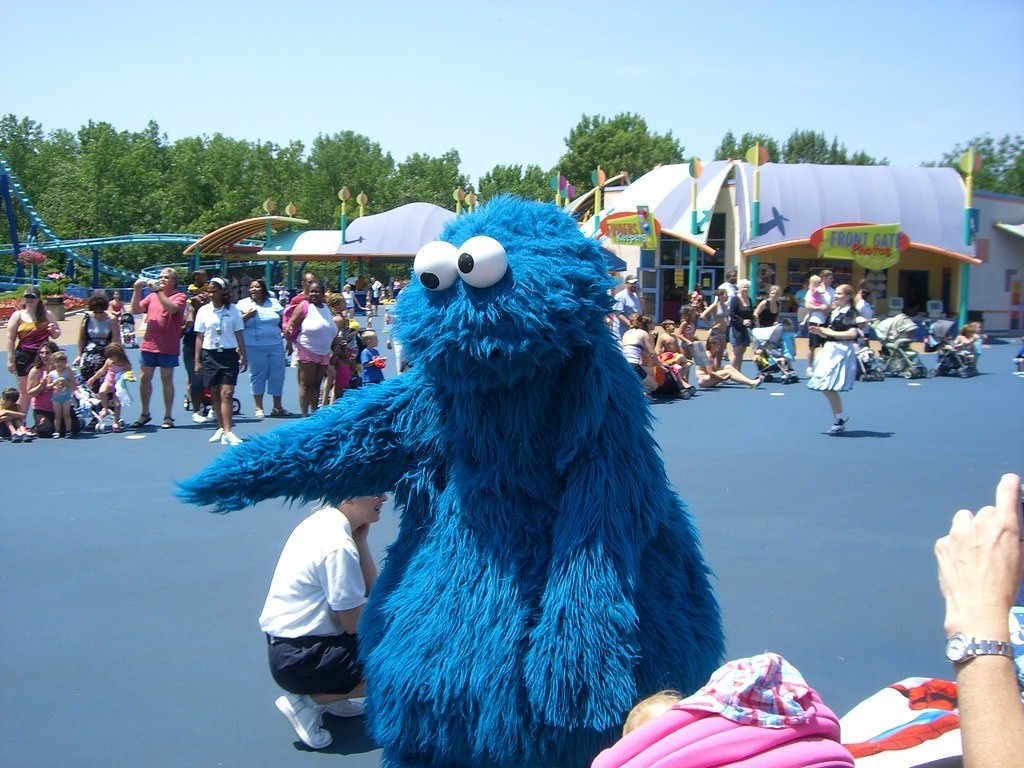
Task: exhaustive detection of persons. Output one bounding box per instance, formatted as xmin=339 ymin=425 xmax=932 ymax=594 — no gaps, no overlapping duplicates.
xmin=613 ymin=274 xmax=697 ymax=399
xmin=853 ymin=279 xmax=878 ymax=348
xmin=753 ymin=285 xmax=796 ymax=379
xmin=700 ymin=270 xmax=755 ymax=372
xmin=856 ymin=315 xmax=869 ymax=342
xmin=1012 ymin=336 xmax=1024 ymax=376
xmin=259 ymin=492 xmax=390 ymax=748
xmin=688 ymin=284 xmax=708 ymax=315
xmin=386 ymin=325 xmax=404 ymax=375
xmin=794 ymin=269 xmax=835 ymax=377
xmin=676 ymin=305 xmax=763 ymax=389
xmin=0 ymin=287 xmax=136 ymax=442
xmin=934 ymin=472 xmax=1024 ymax=768
xmin=806 ymin=284 xmax=860 ymax=432
xmin=108 ymin=267 xmax=187 ymax=429
xmin=389 ymin=275 xmax=402 ymax=299
xmin=232 ymin=270 xmax=387 ymax=419
xmin=180 ymin=269 xmax=248 ymax=446
xmin=954 ymin=322 xmax=988 ymax=370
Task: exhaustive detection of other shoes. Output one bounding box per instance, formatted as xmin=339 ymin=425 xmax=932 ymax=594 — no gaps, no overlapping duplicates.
xmin=753 ymin=376 xmax=765 ymax=388
xmin=807 ymin=370 xmax=813 ymax=378
xmin=830 ymin=416 xmax=849 ymax=431
xmin=827 ymin=428 xmax=845 ymax=435
xmin=11 ymin=408 xmax=124 ymax=442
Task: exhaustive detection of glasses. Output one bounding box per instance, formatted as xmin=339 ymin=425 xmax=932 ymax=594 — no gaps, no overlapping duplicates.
xmin=93 ymin=310 xmax=104 ymax=314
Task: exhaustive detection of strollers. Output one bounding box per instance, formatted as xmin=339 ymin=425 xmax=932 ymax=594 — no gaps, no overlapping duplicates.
xmin=922 ymin=318 xmax=978 ymax=378
xmin=870 ymin=313 xmax=929 ymax=378
xmin=853 ymin=338 xmax=885 ymax=382
xmin=183 ymin=377 xmax=241 ymax=414
xmin=118 ymin=312 xmax=138 ymax=348
xmin=71 ymin=367 xmax=126 ymax=433
xmin=745 ymin=320 xmax=798 ymax=385
xmin=652 ymin=352 xmax=696 ymax=400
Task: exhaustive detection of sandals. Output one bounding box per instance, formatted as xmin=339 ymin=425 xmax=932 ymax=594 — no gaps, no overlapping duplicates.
xmin=162 ymin=417 xmax=175 ymax=429
xmin=271 ymin=408 xmax=292 ymax=416
xmin=131 ymin=413 xmax=152 ymax=427
xmin=254 ymin=410 xmax=264 ymax=419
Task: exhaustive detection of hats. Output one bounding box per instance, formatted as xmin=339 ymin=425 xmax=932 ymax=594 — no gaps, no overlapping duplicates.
xmin=855 ymin=316 xmax=866 ymax=323
xmin=625 ymin=275 xmax=639 ymax=284
xmin=23 ymin=288 xmax=40 ymax=298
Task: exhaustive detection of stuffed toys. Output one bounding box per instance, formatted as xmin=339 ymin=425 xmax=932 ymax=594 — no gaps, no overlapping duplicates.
xmin=173 ymin=191 xmax=728 ymax=767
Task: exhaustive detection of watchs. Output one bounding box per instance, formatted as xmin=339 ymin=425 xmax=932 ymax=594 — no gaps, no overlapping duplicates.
xmin=945 ymin=632 xmax=1014 ymax=664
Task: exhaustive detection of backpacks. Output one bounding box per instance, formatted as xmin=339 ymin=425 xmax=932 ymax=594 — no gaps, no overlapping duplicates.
xmin=281 ymin=300 xmax=309 ymax=342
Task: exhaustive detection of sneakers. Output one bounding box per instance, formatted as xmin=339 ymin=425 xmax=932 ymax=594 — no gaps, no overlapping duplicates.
xmin=221 ymin=431 xmax=243 ymax=445
xmin=327 ymin=699 xmax=364 ymax=717
xmin=208 ymin=428 xmax=224 ymax=442
xmin=207 ymin=409 xmax=218 ymax=420
xmin=193 ymin=411 xmax=207 ymax=423
xmin=275 ymin=693 xmax=332 ymax=749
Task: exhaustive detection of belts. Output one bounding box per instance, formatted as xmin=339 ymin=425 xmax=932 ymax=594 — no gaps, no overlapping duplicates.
xmin=267 ymin=635 xmax=325 ymax=646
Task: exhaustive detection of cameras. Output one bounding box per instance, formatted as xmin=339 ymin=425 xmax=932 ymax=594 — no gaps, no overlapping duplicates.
xmin=1018 ymin=484 xmax=1024 ymax=542
xmin=141 ymin=278 xmax=148 ymax=284
